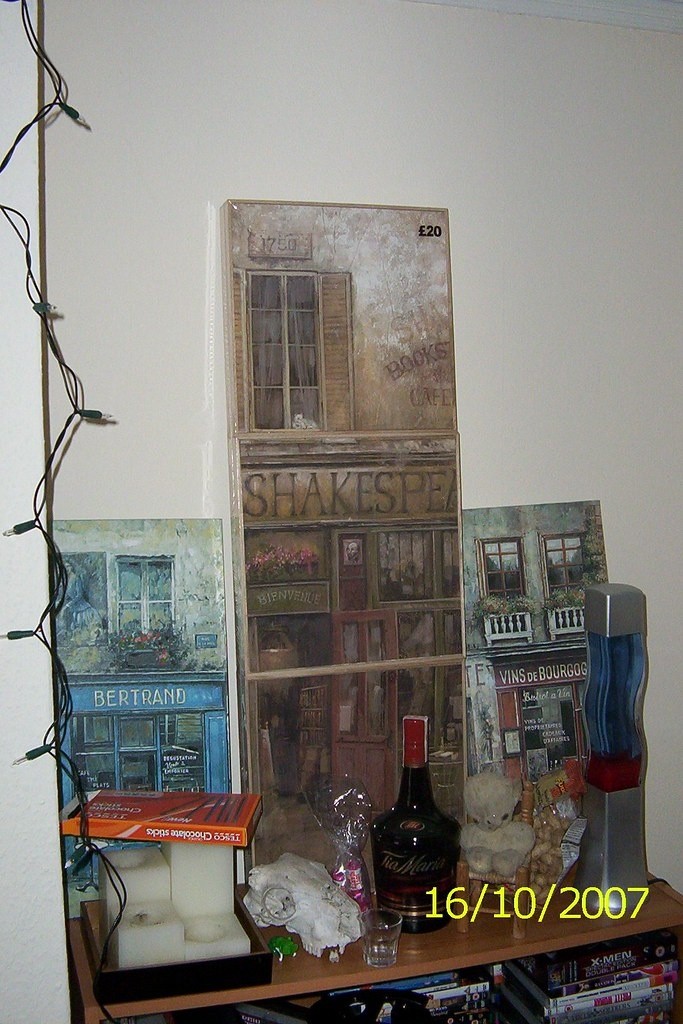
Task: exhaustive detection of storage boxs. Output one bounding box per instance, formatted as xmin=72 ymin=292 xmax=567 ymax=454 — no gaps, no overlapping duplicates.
xmin=80 ymin=891 xmax=273 ymax=1006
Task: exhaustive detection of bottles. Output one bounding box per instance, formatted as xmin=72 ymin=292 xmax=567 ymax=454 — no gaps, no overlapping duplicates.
xmin=372 ymin=719 xmax=461 ymax=935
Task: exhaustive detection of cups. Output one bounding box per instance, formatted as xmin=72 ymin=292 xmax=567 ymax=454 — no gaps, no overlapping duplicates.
xmin=360 ymin=909 xmax=402 ymax=968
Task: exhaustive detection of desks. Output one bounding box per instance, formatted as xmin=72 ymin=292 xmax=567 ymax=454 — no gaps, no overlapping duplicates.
xmin=70 ymin=872 xmax=683 ymax=1024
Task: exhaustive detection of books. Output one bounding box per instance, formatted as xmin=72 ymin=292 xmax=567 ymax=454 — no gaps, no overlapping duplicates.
xmin=496 ymin=930 xmax=679 ymax=1024
xmin=328 ymin=969 xmax=489 ymax=1024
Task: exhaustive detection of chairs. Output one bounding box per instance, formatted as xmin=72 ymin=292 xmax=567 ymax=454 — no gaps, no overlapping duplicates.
xmin=454 ymin=781 xmax=534 ymax=939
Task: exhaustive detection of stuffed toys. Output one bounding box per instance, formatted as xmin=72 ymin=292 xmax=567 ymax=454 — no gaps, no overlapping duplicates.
xmin=459 ymin=772 xmax=537 ymax=878
